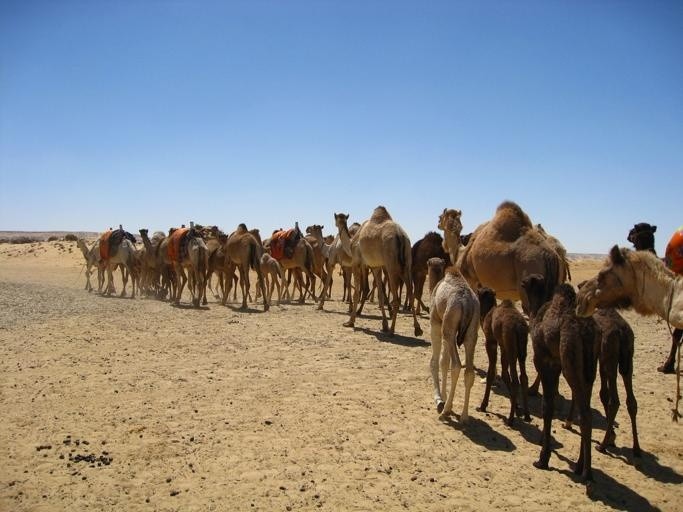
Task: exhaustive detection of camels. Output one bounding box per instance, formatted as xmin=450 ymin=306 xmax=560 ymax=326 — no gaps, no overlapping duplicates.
xmin=575 ymin=243 xmax=683 ymax=330
